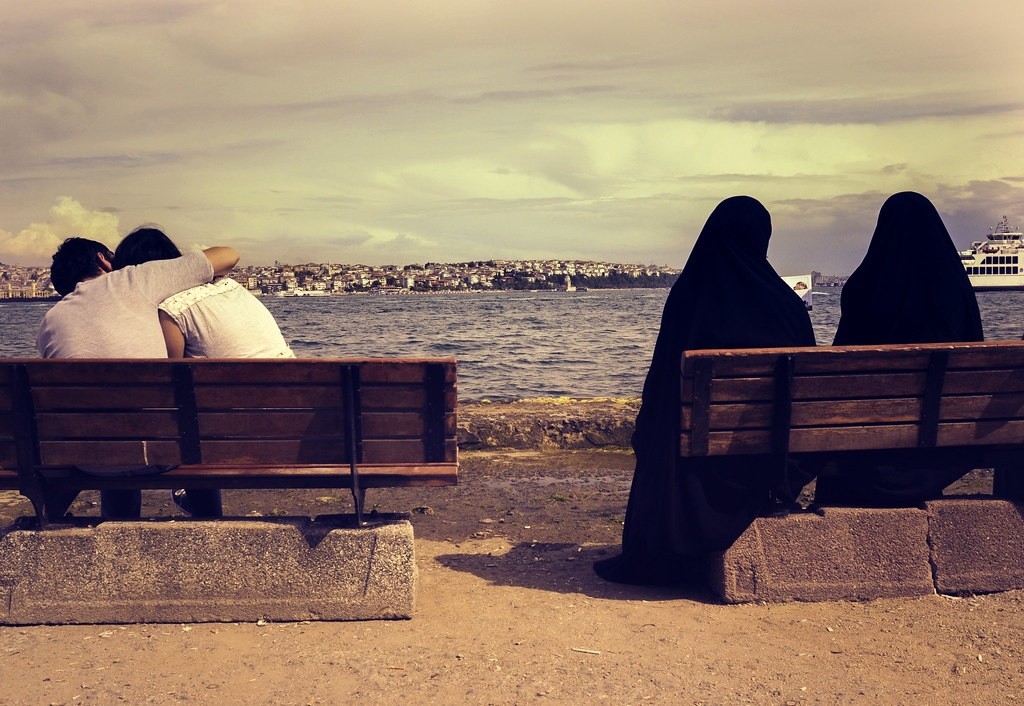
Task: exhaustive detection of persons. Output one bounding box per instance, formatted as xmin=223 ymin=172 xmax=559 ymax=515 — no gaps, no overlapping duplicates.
xmin=18 ymin=237 xmax=241 ymax=521
xmin=112 ymin=224 xmax=299 ymax=518
xmin=813 ymin=190 xmax=985 ymax=509
xmin=588 ymin=195 xmax=819 ymax=591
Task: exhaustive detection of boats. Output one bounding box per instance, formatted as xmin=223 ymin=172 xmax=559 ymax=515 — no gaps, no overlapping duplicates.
xmin=955 ymin=215 xmax=1024 ymax=290
xmin=982 ymin=247 xmax=998 ymax=255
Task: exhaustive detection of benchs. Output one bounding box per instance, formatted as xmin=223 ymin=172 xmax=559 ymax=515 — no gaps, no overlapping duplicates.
xmin=680 ymin=342 xmax=1024 ymax=604
xmin=0 ymin=357 xmax=460 ymax=624
